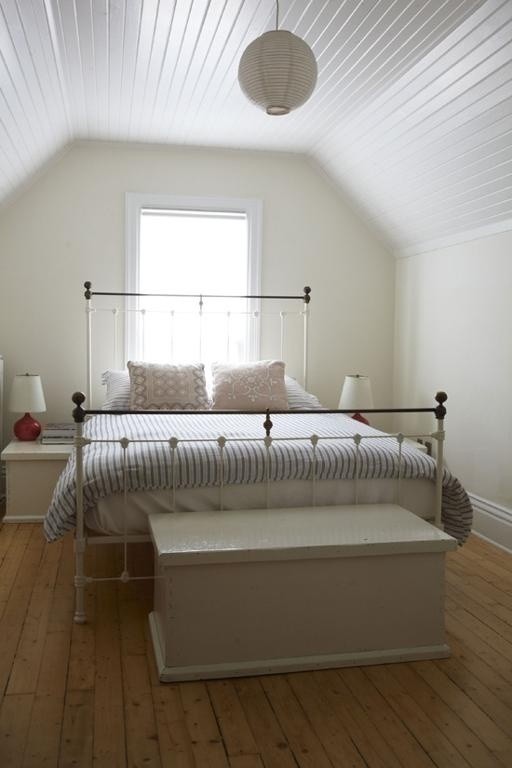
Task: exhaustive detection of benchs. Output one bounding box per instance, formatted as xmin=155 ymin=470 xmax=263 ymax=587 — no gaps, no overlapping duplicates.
xmin=146 ymin=503 xmax=458 ymax=684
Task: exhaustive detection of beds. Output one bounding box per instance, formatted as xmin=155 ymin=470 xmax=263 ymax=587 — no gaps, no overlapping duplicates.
xmin=42 ymin=281 xmax=474 ymax=625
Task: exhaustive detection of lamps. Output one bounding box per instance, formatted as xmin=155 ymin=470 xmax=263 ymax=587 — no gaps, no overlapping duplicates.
xmin=337 ymin=374 xmax=373 ymax=425
xmin=8 ymin=371 xmax=47 ymax=441
xmin=236 ymin=0 xmax=318 ymax=117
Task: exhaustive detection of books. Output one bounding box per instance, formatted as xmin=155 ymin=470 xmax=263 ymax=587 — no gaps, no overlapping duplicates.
xmin=39 ymin=434 xmax=75 ymax=445
xmin=43 ymin=423 xmax=77 ymax=436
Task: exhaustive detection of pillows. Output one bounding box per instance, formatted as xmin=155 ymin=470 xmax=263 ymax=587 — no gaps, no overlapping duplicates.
xmin=101 ymin=359 xmax=322 ymax=411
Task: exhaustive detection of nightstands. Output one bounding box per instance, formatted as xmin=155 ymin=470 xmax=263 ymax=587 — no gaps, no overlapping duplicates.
xmin=1 ymin=437 xmax=75 ymax=525
